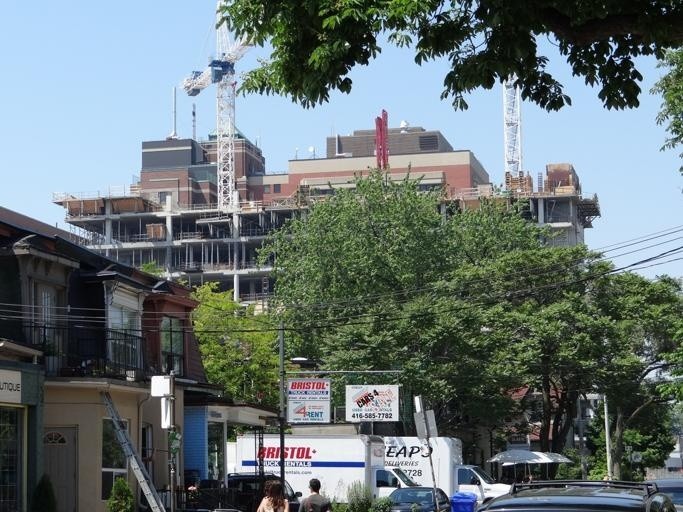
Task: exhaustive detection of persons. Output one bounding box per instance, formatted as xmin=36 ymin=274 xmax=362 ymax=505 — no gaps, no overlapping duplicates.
xmin=256 ymin=480 xmax=290 ymax=512
xmin=298 ymin=479 xmax=332 ymax=512
xmin=187 ymin=481 xmax=197 ymax=501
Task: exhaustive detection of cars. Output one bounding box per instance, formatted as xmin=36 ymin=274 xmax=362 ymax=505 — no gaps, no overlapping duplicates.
xmin=386 ymin=485 xmax=449 ymax=512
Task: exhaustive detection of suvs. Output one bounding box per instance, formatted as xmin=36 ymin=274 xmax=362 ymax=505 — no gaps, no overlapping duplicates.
xmin=222 ymin=471 xmax=302 ymax=512
xmin=478 ymin=479 xmax=674 ymax=512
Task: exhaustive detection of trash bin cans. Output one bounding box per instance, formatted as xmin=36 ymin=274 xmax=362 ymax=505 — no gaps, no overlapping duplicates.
xmin=451 ymin=493 xmax=478 ymax=512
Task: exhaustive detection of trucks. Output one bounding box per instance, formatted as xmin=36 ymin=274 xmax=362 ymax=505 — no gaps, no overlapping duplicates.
xmin=224 ymin=433 xmax=418 ymax=504
xmin=381 ymin=437 xmax=514 ymax=504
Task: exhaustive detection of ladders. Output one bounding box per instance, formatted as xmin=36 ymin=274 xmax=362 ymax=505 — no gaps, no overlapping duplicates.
xmin=99 ymin=391 xmax=167 ymax=512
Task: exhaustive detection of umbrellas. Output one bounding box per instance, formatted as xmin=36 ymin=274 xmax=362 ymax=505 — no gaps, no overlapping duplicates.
xmin=486 ymin=449 xmax=576 ymax=480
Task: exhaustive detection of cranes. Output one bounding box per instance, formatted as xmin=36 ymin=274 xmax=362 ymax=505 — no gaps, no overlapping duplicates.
xmin=180 ymin=0 xmax=264 ymax=217
